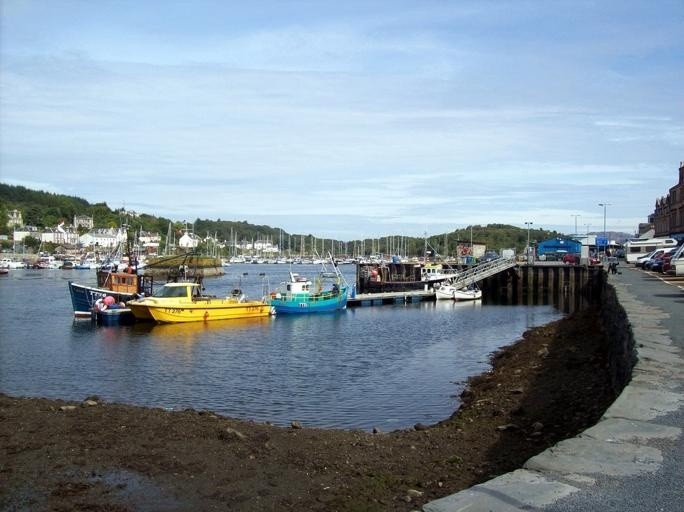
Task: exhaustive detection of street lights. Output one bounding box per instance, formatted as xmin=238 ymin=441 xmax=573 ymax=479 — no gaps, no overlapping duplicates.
xmin=584 ymin=222 xmax=593 ymax=244
xmin=570 ymin=215 xmax=581 ymax=234
xmin=600 ymin=202 xmax=611 ymax=233
xmin=523 ymin=221 xmax=532 ymax=249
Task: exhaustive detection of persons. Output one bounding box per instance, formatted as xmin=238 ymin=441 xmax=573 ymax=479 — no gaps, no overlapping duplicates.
xmin=606 ymin=255 xmax=618 ymax=274
xmin=124 ymin=264 xmax=132 ymax=274
xmin=392 ymin=253 xmax=401 ymax=275
xmin=332 ymin=284 xmax=339 ymax=294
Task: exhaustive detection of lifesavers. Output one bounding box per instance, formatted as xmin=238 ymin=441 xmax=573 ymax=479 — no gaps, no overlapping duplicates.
xmin=95 ymin=299 xmax=108 ymax=311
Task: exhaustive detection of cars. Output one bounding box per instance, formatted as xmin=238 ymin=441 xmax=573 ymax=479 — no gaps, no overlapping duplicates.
xmin=636 ymin=243 xmax=684 ymax=275
xmin=564 ymin=253 xmax=600 ymax=266
